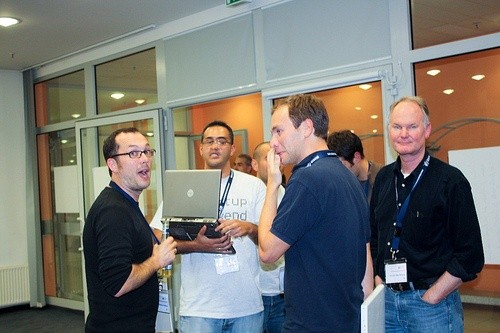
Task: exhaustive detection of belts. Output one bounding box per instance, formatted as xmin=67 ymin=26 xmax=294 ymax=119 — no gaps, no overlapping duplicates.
xmin=387 ymin=280 xmax=429 ymax=291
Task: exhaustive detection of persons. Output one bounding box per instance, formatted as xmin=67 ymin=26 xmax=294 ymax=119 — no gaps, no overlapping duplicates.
xmin=369 ymin=96 xmax=484 ymax=333
xmin=252 ymin=142 xmax=285 ymax=333
xmin=84 ymin=127 xmax=178 ymax=333
xmin=149 ymin=120 xmax=266 ymax=333
xmin=327 ymin=130 xmax=386 ymax=205
xmin=259 ymin=93 xmax=373 ymax=333
xmin=233 ymin=154 xmax=253 ymax=173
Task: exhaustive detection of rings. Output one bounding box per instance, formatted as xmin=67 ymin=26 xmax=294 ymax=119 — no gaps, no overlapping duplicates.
xmin=219 ymin=248 xmax=221 ymax=251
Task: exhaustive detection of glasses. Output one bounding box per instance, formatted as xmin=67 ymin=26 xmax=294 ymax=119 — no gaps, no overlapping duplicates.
xmin=110 ymin=148 xmax=157 ymax=159
xmin=201 ymin=137 xmax=232 ymax=145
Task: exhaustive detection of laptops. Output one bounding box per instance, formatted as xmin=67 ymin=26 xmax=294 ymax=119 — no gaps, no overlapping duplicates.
xmin=160 ymin=169 xmax=236 ymax=255
